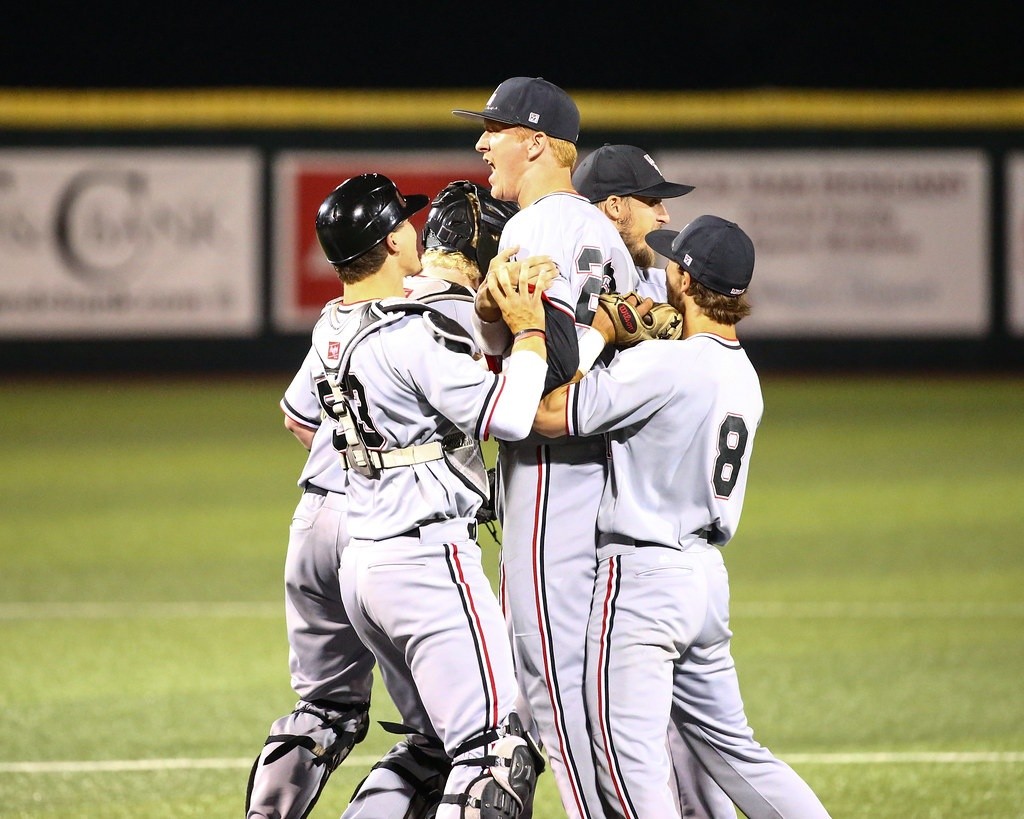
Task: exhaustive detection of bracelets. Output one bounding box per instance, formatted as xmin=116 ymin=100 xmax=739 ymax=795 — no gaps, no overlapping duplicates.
xmin=513 ymin=332 xmax=546 ymax=342
xmin=514 ymin=328 xmax=546 ymax=339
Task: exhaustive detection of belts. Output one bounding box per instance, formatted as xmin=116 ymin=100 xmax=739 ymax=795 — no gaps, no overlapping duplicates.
xmin=635 ymin=528 xmax=713 ymax=551
xmin=401 ymin=518 xmax=476 ymax=540
xmin=304 ymin=484 xmax=328 ymax=496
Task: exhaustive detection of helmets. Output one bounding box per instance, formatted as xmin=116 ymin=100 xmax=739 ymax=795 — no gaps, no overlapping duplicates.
xmin=420 ymin=179 xmax=521 ymax=279
xmin=314 ymin=174 xmax=429 ymax=270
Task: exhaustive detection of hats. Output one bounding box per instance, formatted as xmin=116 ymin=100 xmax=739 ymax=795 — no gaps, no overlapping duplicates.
xmin=571 ymin=142 xmax=695 ymax=204
xmin=645 ymin=215 xmax=754 ymax=297
xmin=452 ymin=77 xmax=580 ymax=146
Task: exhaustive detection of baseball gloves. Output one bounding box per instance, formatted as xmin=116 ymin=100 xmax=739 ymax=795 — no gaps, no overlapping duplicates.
xmin=598 ymin=286 xmax=683 ymax=352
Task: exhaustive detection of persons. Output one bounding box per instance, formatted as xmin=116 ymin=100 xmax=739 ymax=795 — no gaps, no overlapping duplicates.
xmin=533 ymin=215 xmax=832 ymax=819
xmin=246 ymin=179 xmax=522 ymax=819
xmin=452 ymin=75 xmax=738 ymax=819
xmin=311 ymin=175 xmax=547 ymax=819
